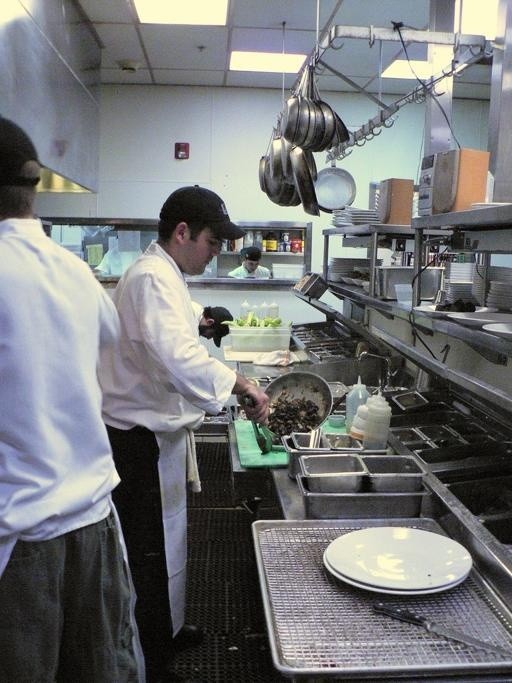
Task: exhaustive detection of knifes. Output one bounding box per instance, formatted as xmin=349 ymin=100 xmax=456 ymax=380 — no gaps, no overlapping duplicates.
xmin=372 ymin=601 xmax=512 ymax=658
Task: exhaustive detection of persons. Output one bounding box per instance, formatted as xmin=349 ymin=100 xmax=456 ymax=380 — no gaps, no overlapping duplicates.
xmin=100 ymin=184 xmax=269 ymax=651
xmin=0 ymin=116 xmax=148 ymax=683
xmin=190 ymin=301 xmax=233 ymax=348
xmin=228 ymin=247 xmax=272 ymax=280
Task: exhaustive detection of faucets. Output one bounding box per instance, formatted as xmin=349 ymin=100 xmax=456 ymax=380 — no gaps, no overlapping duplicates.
xmin=358 ymin=352 xmax=393 ymax=389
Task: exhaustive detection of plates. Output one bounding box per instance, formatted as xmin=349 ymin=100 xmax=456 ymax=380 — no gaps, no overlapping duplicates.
xmin=332 ymin=210 xmax=352 ymax=228
xmin=482 ymin=323 xmax=512 ymax=341
xmin=351 ymin=279 xmax=363 ymax=286
xmin=413 ymin=305 xmax=499 ymax=318
xmin=344 ymin=210 xmax=380 ymax=225
xmin=474 ymin=264 xmax=512 ymax=307
xmin=362 ymin=282 xmax=369 ymax=293
xmin=448 ymin=281 xmax=474 ymax=304
xmin=486 ymin=280 xmax=512 ymax=311
xmin=328 ymin=257 xmax=384 ymax=283
xmin=450 ymin=263 xmax=480 ymax=282
xmin=341 ymin=277 xmax=353 ymax=285
xmin=471 ymin=202 xmax=512 ymax=209
xmin=322 ymin=525 xmax=473 ymax=596
xmin=446 ymin=312 xmax=512 ymax=327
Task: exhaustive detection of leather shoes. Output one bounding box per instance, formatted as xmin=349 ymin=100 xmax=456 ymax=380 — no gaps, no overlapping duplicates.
xmin=172 ymin=624 xmax=203 ymax=645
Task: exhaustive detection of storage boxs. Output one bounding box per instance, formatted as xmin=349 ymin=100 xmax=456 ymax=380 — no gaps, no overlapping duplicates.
xmin=230 ymin=328 xmax=292 ymax=351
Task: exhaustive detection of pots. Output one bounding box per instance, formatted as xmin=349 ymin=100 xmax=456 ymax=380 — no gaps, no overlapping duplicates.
xmin=313 ymin=160 xmax=356 ymax=214
xmin=289 ymin=146 xmax=320 ymax=216
xmin=281 ymin=135 xmax=294 ymax=178
xmin=263 ymin=156 xmax=294 ymax=206
xmin=288 ymin=191 xmax=301 ymax=206
xmin=244 ymin=371 xmax=333 ymax=439
xmin=305 ymin=151 xmax=319 ymax=181
xmin=259 ymin=128 xmax=275 ymax=192
xmin=281 ymin=64 xmax=350 ymax=152
xmin=269 ymin=119 xmax=283 ymax=181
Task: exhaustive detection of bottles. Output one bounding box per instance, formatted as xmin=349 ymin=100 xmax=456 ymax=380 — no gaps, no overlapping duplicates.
xmin=345 ymin=375 xmax=370 ymax=434
xmin=221 ymin=239 xmax=231 ymax=251
xmin=363 ymin=397 xmax=393 ymax=450
xmin=244 ymin=230 xmax=253 ymax=248
xmin=277 ymin=242 xmax=285 ymax=251
xmin=235 ymin=237 xmax=243 ymax=252
xmin=350 ymin=391 xmax=385 ymax=440
xmin=254 ymin=231 xmax=263 ymax=251
xmin=266 ymin=230 xmax=278 ymax=252
xmin=290 ymin=239 xmax=302 ymax=252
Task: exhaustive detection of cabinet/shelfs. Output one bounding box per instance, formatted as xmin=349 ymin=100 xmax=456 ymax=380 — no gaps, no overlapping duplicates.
xmin=321 ymin=204 xmax=512 ymax=413
xmin=217 ymin=228 xmax=304 ymax=278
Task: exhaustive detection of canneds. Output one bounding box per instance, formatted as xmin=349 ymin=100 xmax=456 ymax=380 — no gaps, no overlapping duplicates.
xmin=278 ymin=242 xmax=284 ymax=253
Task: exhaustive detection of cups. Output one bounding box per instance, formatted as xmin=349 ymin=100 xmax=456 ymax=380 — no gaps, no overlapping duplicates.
xmin=393 ymin=284 xmax=414 ymax=304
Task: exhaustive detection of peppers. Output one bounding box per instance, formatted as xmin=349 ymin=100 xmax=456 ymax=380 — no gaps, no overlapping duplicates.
xmin=220 ymin=312 xmax=293 ymax=327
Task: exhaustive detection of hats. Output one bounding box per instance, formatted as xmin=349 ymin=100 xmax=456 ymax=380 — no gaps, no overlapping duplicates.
xmin=160 ymin=185 xmax=248 ymax=240
xmin=241 ymin=247 xmax=261 ymax=261
xmin=211 ymin=307 xmax=233 ymax=347
xmin=0 ymin=117 xmax=41 ymax=186
